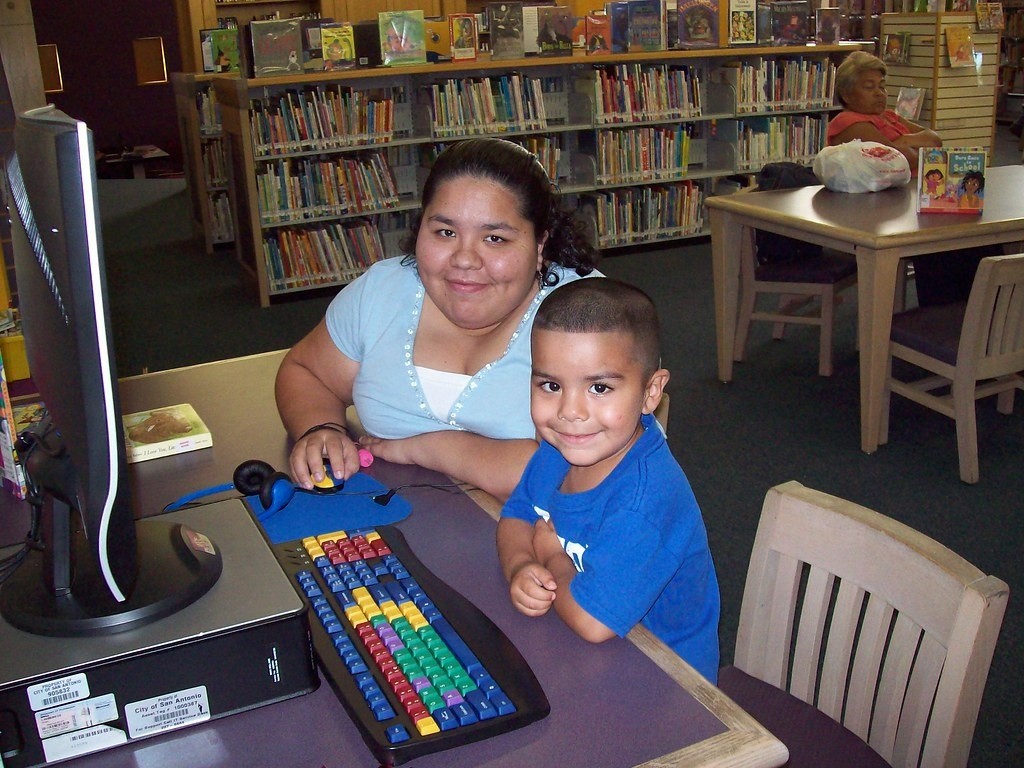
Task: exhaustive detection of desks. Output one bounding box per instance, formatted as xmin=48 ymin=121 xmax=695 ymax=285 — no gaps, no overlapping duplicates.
xmin=704 ymin=164 xmax=1024 ymax=454
xmin=0 ymin=348 xmax=790 ymax=768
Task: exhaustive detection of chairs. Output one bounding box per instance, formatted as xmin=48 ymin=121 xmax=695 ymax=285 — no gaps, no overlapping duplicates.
xmin=877 ymin=252 xmax=1024 ymax=485
xmin=733 ymin=184 xmax=858 ymax=377
xmin=734 ymin=481 xmax=1009 ymax=768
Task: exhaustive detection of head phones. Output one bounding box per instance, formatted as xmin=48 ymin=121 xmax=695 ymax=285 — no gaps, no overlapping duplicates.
xmin=163 ymin=459 xmax=295 ymax=523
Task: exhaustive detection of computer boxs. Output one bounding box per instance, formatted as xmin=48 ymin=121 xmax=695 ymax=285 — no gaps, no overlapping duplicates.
xmin=0 ymin=493 xmax=321 ymax=768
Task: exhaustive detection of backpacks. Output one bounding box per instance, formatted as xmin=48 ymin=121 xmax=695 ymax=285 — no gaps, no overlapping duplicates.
xmin=755 ymin=163 xmax=822 ymax=264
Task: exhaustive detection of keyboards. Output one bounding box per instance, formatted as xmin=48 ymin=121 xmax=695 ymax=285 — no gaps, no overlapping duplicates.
xmin=269 ymin=524 xmax=549 ymax=767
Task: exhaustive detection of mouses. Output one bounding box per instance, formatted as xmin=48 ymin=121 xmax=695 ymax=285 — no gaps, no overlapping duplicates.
xmin=308 ymin=458 xmax=345 ymax=495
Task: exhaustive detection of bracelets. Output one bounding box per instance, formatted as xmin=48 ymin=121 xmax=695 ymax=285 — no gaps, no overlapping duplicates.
xmin=298 ymin=423 xmax=348 ymax=441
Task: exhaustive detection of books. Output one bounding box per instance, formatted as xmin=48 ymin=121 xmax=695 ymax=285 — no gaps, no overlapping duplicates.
xmin=585 ymin=0 xmax=666 ymax=56
xmin=198 ymin=28 xmax=240 ymax=74
xmin=475 ymin=2 xmax=573 ymax=61
xmin=916 ymin=146 xmax=987 ymax=214
xmin=250 ymin=18 xmax=304 ymax=77
xmin=448 ymin=14 xmax=477 ymax=62
xmin=719 ymin=57 xmax=836 ymax=171
xmin=581 ymin=63 xmax=706 ymax=245
xmin=677 ymin=0 xmax=840 ymax=49
xmin=248 ymin=83 xmax=401 ymax=291
xmin=196 ymin=87 xmax=236 ymax=240
xmin=122 ymin=404 xmax=213 ymax=464
xmin=377 ymin=10 xmax=426 ymax=66
xmin=417 ymin=71 xmax=560 ymax=190
xmin=319 ymin=22 xmax=356 ymax=71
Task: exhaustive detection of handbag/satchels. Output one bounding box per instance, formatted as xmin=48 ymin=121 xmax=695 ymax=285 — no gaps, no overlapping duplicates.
xmin=812 ymin=140 xmax=911 ymax=194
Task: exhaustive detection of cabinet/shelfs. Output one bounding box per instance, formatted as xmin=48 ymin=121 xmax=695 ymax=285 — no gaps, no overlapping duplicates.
xmin=218 ymin=43 xmax=878 ymax=309
xmin=170 ymin=71 xmax=239 ymax=258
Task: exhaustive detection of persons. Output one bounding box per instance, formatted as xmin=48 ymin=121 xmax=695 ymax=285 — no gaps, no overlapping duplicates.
xmin=274 ymin=135 xmax=606 ymax=503
xmin=826 ymin=51 xmax=943 ymax=177
xmin=496 ymin=277 xmax=721 ymax=685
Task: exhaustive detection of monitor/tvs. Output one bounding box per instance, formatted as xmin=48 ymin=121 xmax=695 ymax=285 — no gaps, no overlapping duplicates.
xmin=0 ymin=102 xmax=223 ymax=645
